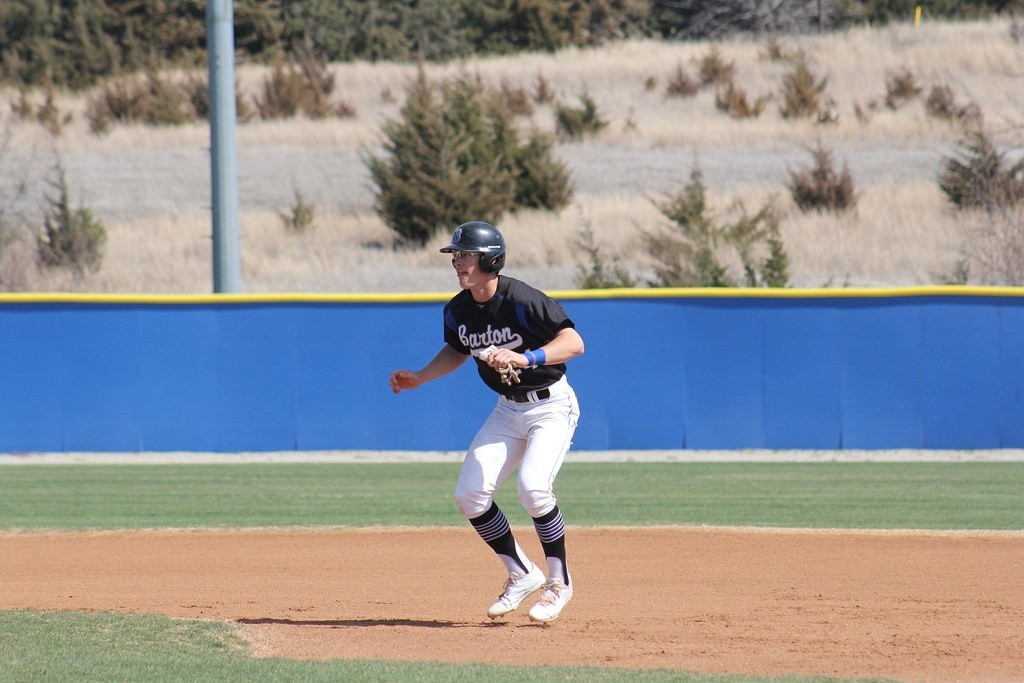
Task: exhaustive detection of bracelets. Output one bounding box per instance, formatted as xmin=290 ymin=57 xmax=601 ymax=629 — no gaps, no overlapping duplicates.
xmin=524 ymin=348 xmax=545 ymax=365
xmin=524 ymin=349 xmax=537 ymax=369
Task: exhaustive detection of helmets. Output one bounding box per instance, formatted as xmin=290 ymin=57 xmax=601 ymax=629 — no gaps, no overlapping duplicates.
xmin=439 ymin=221 xmax=506 ymax=273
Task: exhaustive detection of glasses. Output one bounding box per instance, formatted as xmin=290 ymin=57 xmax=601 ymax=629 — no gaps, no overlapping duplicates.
xmin=451 ymin=250 xmax=482 ymax=258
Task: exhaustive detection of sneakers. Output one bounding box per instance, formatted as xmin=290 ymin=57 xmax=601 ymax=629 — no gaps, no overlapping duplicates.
xmin=487 ymin=561 xmax=547 ymax=618
xmin=528 ymin=575 xmax=573 ymax=622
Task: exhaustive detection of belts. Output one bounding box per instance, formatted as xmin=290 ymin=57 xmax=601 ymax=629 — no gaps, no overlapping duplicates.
xmin=500 ymin=388 xmax=550 ymax=403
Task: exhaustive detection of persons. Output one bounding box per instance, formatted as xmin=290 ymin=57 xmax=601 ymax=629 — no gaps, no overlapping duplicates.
xmin=390 ymin=221 xmax=585 ymax=624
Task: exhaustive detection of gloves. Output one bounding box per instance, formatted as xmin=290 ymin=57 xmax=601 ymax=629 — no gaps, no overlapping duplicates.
xmin=479 ymin=344 xmax=521 ymax=386
